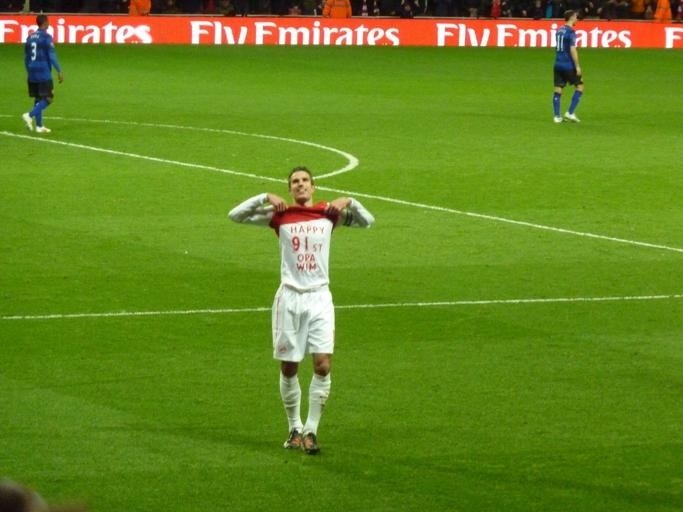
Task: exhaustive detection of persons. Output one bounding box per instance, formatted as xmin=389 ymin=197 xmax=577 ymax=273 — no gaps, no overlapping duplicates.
xmin=351 ymin=0 xmax=683 ymax=20
xmin=323 ymin=0 xmax=352 ymax=19
xmin=1 ymin=1 xmax=130 ymax=13
xmin=150 ymin=1 xmax=323 ymax=16
xmin=549 ymin=10 xmax=586 ymax=124
xmin=227 ymin=166 xmax=375 ymax=453
xmin=19 ymin=15 xmax=63 ymax=134
xmin=128 ymin=1 xmax=152 ymax=16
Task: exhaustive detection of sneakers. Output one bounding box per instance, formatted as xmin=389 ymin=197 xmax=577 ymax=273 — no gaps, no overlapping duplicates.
xmin=302 ymin=433 xmax=320 ymax=454
xmin=553 ymin=112 xmax=581 ymax=124
xmin=36 ymin=126 xmax=51 ymax=133
xmin=23 ymin=113 xmax=33 ymax=131
xmin=284 ymin=429 xmax=302 ymax=448
